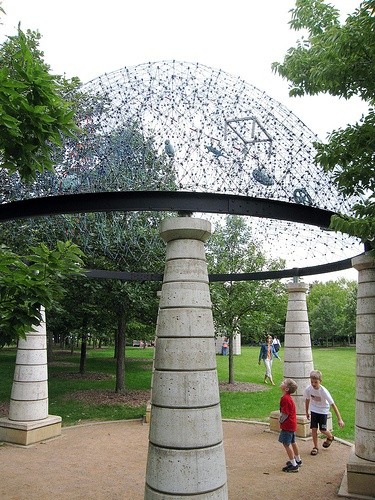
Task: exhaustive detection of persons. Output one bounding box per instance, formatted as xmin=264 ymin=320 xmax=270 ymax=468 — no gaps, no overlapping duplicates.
xmin=278 ymin=378 xmax=303 ymax=473
xmin=259 ymin=335 xmax=281 ymax=386
xmin=303 ymin=369 xmax=345 ymax=455
xmin=139 ymin=340 xmax=143 ymax=348
xmin=271 ymin=335 xmax=282 ymax=359
xmin=222 ymin=336 xmax=229 ymax=356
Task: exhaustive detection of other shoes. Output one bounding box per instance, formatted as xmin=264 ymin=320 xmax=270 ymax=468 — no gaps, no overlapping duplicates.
xmin=271 ymin=381 xmax=275 ymax=385
xmin=264 ymin=380 xmax=267 ymax=384
xmin=282 ymin=461 xmax=299 ymax=472
xmin=286 ymin=459 xmax=302 ymax=466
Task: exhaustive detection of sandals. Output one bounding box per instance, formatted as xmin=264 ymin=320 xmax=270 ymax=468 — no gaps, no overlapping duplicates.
xmin=323 ymin=436 xmax=334 ymax=447
xmin=311 ymin=448 xmax=318 ymax=455
